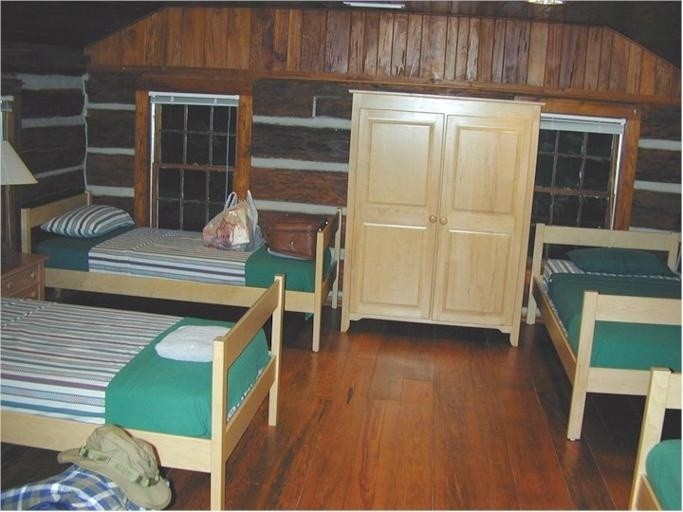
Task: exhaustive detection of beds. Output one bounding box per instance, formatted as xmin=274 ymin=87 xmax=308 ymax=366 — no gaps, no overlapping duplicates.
xmin=628 ymin=366 xmax=681 ymax=510
xmin=18 ymin=189 xmax=341 ymax=353
xmin=0 ymin=283 xmax=287 ymax=509
xmin=525 ymin=224 xmax=681 ymax=441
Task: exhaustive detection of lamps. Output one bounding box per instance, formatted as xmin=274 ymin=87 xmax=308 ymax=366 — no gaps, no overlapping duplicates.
xmin=0 ymin=141 xmax=38 ymax=186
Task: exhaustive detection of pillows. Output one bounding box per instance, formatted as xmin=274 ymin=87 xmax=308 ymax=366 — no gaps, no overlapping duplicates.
xmin=563 ymin=248 xmax=679 ymax=276
xmin=38 ymin=202 xmax=135 ymax=238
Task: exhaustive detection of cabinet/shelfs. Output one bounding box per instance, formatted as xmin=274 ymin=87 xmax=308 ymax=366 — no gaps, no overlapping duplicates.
xmin=341 ymin=89 xmax=544 ymax=350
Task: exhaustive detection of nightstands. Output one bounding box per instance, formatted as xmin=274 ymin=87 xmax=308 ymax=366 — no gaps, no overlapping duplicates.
xmin=1 ymin=253 xmax=48 ymax=300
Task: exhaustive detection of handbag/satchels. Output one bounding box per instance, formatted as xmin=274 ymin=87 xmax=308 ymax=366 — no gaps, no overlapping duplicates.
xmin=264 ymin=216 xmax=329 ymax=259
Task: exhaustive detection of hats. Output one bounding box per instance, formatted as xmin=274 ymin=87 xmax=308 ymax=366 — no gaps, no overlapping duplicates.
xmin=57 ymin=425 xmax=173 ymax=509
